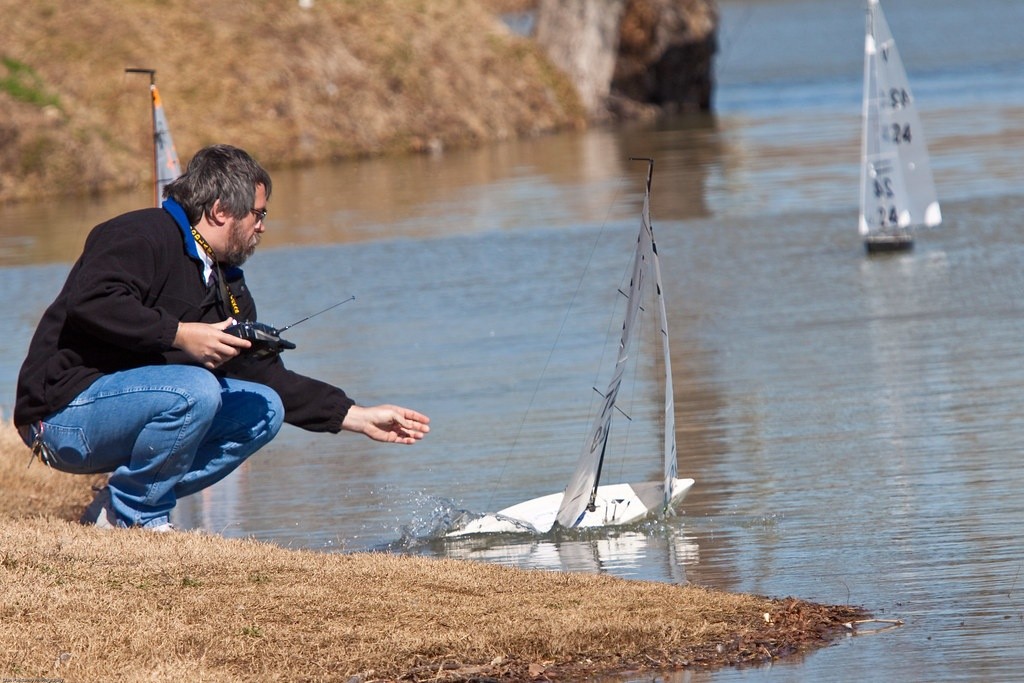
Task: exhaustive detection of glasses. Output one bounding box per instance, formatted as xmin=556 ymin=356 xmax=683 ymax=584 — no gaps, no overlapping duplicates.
xmin=251 ymin=208 xmax=267 ymax=222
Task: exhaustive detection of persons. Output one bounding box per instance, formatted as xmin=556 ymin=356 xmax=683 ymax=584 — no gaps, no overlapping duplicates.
xmin=618 ymin=0 xmax=717 ymax=107
xmin=13 ymin=144 xmax=431 ymax=531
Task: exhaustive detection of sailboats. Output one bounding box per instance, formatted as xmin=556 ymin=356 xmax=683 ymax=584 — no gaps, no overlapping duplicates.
xmin=435 ymin=157 xmax=696 ymax=539
xmin=852 ymin=0 xmax=945 ymax=249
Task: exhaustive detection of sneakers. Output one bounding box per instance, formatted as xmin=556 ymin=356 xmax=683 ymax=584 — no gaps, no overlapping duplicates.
xmin=80 ymin=486 xmax=174 ymax=532
